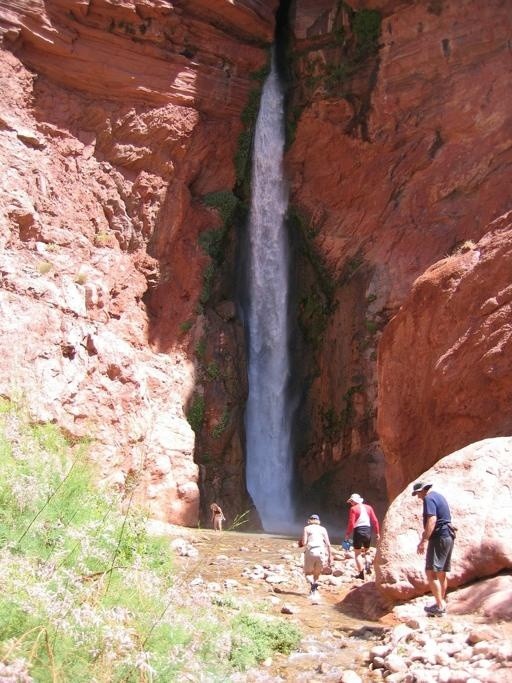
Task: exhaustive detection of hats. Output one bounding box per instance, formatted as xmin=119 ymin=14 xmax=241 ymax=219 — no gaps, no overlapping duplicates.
xmin=310 ymin=515 xmax=320 ymax=519
xmin=412 ymin=482 xmax=432 ymax=496
xmin=347 ymin=494 xmax=364 ymax=504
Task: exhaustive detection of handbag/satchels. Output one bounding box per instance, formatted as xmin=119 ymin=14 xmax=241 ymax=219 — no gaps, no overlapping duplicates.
xmin=429 ymin=524 xmax=442 ymax=538
xmin=342 ymin=541 xmax=350 ymax=550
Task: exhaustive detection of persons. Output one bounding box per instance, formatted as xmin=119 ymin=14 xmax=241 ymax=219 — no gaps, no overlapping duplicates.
xmin=411 ymin=481 xmax=456 ymax=615
xmin=345 ymin=493 xmax=380 ymax=580
xmin=210 ymin=503 xmax=225 ymax=532
xmin=298 ymin=514 xmax=333 ymax=592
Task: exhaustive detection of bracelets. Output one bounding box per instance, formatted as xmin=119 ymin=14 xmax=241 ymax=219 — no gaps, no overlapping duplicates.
xmin=376 ymin=536 xmax=378 ymax=538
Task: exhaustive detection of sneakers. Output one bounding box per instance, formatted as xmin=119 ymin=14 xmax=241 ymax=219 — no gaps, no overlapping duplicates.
xmin=365 ymin=562 xmax=371 ymax=574
xmin=424 ymin=604 xmax=447 ymax=616
xmin=356 ymin=571 xmax=364 ymax=579
